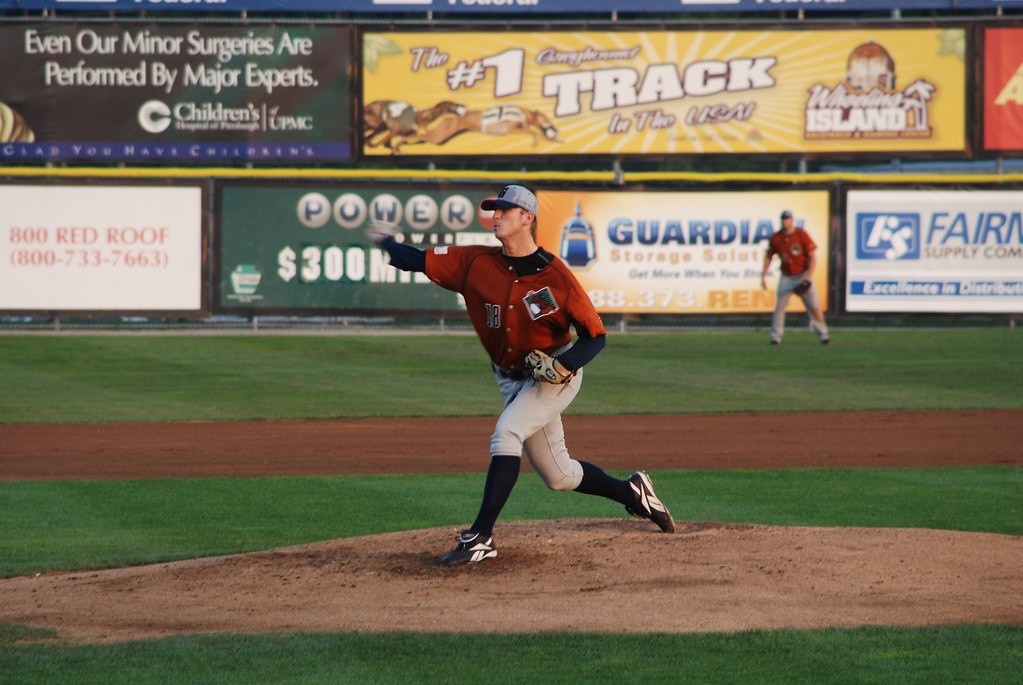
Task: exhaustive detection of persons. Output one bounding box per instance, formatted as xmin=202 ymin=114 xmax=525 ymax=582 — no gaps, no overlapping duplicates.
xmin=367 ymin=186 xmax=676 ymax=566
xmin=761 ymin=210 xmax=832 ymax=346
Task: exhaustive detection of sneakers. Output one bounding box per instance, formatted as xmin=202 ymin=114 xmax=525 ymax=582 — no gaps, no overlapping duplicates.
xmin=443 ymin=530 xmax=497 ymax=569
xmin=627 ymin=472 xmax=675 ymax=533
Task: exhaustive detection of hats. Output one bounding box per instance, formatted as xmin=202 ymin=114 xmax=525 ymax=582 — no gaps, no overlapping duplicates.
xmin=481 ymin=184 xmax=538 ymax=216
xmin=781 ymin=211 xmax=792 ymax=218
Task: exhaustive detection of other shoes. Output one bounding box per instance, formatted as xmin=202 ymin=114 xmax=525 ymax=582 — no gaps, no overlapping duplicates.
xmin=770 ymin=340 xmax=778 ymax=345
xmin=821 ymin=340 xmax=831 ymax=345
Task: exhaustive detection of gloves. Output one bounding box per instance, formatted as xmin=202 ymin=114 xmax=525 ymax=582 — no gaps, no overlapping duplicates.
xmin=525 ymin=349 xmax=568 ymax=386
xmin=792 ymin=279 xmax=812 ymax=296
